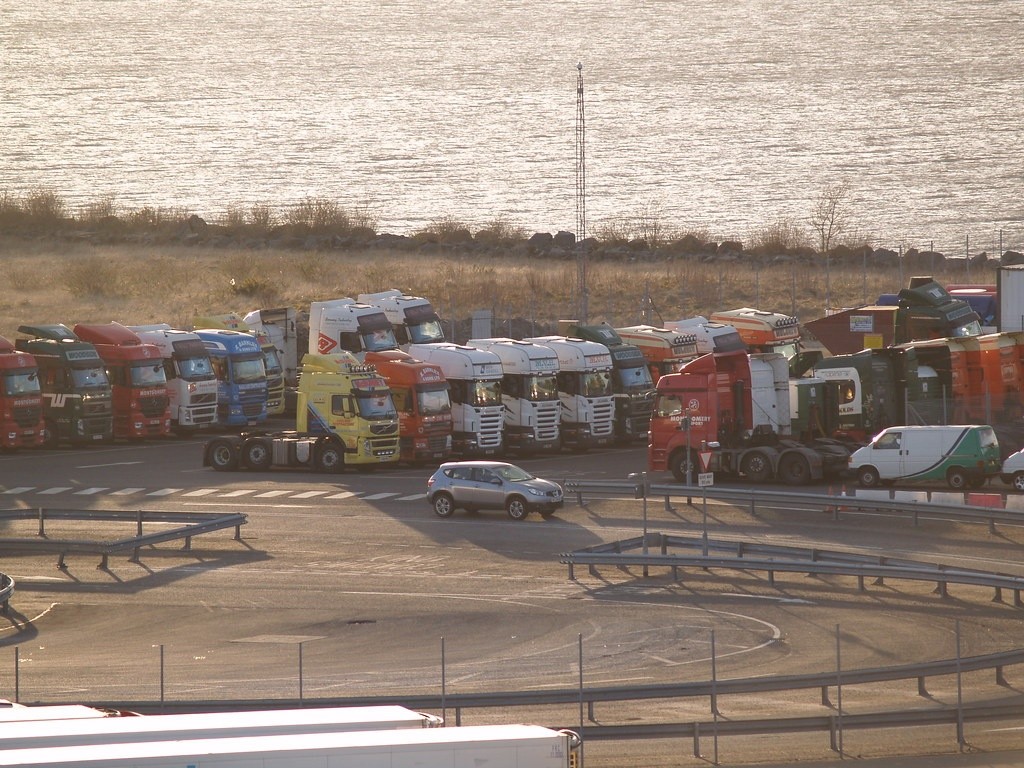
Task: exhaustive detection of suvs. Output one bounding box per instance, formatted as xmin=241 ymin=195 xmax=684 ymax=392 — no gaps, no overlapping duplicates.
xmin=427 ymin=461 xmax=563 ymax=520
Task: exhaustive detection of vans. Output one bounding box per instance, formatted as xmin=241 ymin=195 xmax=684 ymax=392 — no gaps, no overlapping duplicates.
xmin=1001 ymin=447 xmax=1024 ymax=491
xmin=849 ymin=424 xmax=1001 ymax=490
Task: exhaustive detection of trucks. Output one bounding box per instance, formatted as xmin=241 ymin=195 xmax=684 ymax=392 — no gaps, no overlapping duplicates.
xmin=203 ymin=353 xmax=400 ymax=472
xmin=567 ymin=322 xmax=656 ymax=444
xmin=523 ymin=335 xmax=615 ymax=450
xmin=466 ymin=337 xmax=561 ymax=458
xmin=407 ymin=343 xmax=507 ymax=459
xmin=618 ymin=276 xmax=1024 ymax=484
xmin=360 ymin=349 xmax=452 ymax=466
xmin=0 ymin=288 xmax=451 ymax=452
